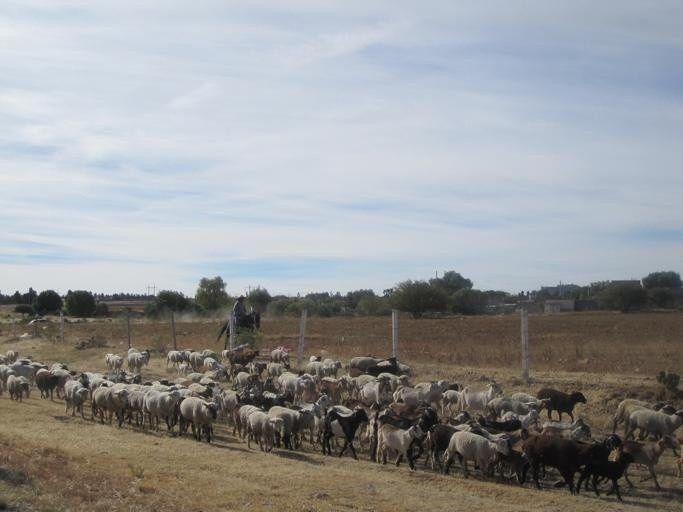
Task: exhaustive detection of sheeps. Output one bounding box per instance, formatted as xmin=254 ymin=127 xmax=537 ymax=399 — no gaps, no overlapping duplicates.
xmin=0 ymin=343 xmax=683 ymax=502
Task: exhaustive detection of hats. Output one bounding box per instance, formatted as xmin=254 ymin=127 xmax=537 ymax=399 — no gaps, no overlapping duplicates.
xmin=236 ymin=295 xmax=246 ymax=300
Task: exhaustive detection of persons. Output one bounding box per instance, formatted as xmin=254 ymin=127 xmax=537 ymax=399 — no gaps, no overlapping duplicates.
xmin=232 ymin=294 xmax=247 ymax=334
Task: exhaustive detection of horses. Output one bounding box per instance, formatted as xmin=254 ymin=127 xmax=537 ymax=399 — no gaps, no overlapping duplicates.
xmin=217 ymin=310 xmax=262 ymax=350
